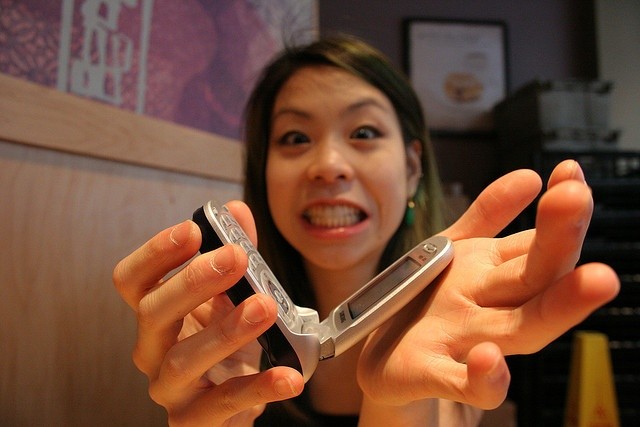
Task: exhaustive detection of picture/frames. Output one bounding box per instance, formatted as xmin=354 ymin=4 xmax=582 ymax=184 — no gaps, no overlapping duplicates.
xmin=400 ymin=13 xmax=510 ymax=138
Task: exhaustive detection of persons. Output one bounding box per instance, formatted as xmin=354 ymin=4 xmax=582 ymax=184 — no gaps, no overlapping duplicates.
xmin=111 ymin=33 xmax=622 ymax=425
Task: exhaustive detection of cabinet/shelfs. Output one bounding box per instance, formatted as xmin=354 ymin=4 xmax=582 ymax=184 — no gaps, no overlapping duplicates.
xmin=489 ymin=147 xmax=639 ymax=426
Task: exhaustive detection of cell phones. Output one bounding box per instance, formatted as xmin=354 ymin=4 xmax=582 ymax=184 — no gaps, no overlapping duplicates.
xmin=192 ymin=201 xmax=455 ymax=384
